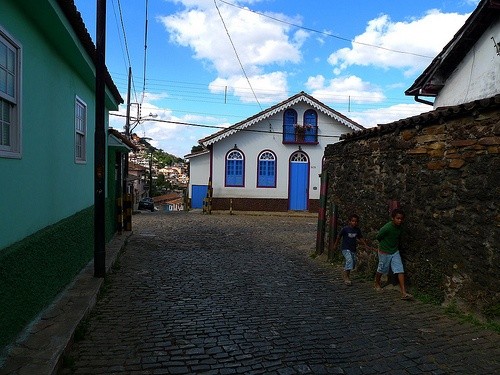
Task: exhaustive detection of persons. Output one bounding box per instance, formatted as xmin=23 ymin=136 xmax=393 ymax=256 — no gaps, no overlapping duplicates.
xmin=372 ymin=206 xmax=414 ymax=299
xmin=335 ymin=213 xmax=371 ymax=286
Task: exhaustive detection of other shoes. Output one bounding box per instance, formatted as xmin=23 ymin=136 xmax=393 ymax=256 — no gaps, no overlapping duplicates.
xmin=374 ymin=282 xmax=384 ymax=292
xmin=401 ymin=292 xmax=414 ymax=299
xmin=343 ymin=274 xmax=352 ymax=285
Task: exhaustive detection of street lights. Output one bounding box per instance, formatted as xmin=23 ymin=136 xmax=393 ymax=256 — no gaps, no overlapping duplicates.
xmin=122 ymin=112 xmax=159 ymax=214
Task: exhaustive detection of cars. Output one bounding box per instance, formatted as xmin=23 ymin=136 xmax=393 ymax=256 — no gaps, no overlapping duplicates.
xmin=138 ymin=197 xmax=154 ymax=212
